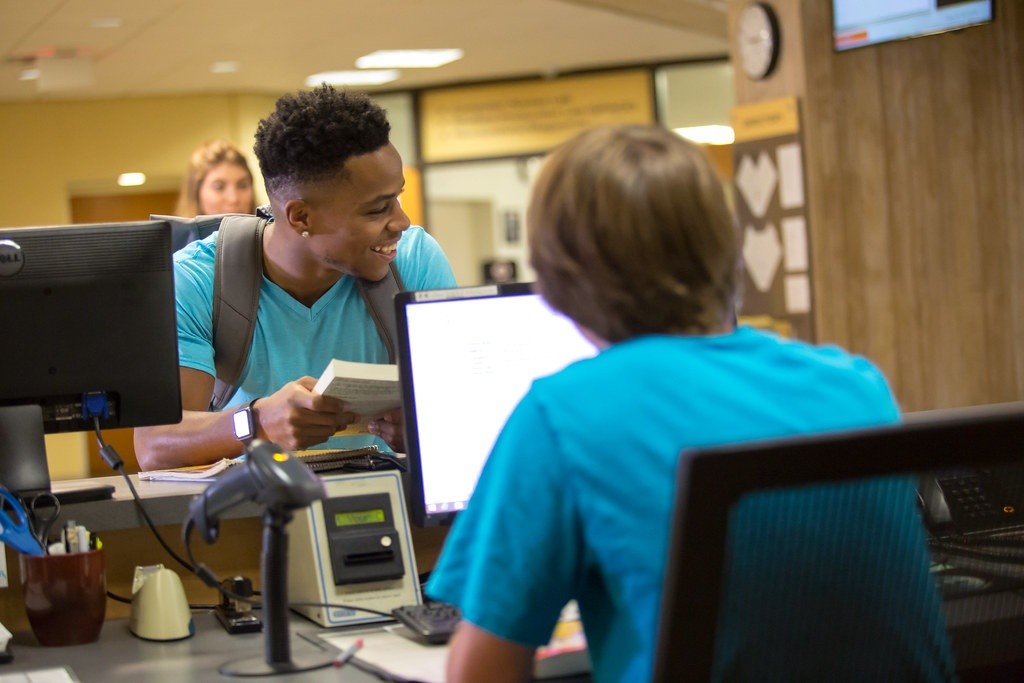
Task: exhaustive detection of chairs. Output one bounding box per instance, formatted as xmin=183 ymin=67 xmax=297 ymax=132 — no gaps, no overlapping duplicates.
xmin=654 ymin=399 xmax=1024 ymax=683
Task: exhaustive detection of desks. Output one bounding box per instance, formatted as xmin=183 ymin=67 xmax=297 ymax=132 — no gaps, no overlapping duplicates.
xmin=0 ymin=543 xmax=1024 ymax=683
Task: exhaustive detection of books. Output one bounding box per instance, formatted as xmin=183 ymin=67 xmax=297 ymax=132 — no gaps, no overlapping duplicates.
xmin=312 ymin=359 xmax=402 ymax=430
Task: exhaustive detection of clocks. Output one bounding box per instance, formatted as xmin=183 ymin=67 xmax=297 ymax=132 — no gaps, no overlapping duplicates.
xmin=733 ymin=3 xmax=780 ymax=81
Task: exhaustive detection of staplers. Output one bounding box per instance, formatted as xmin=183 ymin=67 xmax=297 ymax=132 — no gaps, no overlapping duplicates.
xmin=213 ymin=577 xmax=263 ymax=635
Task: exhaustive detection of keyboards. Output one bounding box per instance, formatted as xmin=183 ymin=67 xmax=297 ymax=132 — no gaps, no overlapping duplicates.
xmin=392 ymin=602 xmax=462 ymax=645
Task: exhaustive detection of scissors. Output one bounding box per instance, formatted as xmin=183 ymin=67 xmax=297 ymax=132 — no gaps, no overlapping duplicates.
xmin=1 ymin=485 xmax=45 ymax=556
xmin=4 ymin=491 xmax=61 ymax=554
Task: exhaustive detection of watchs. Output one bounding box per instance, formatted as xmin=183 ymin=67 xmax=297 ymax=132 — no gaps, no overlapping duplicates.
xmin=232 ymin=397 xmax=260 ymax=448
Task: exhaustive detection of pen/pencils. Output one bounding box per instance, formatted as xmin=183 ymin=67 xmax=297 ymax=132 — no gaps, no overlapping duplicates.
xmin=333 ymin=639 xmax=363 ymax=668
xmin=63 ymin=520 xmax=102 ymax=553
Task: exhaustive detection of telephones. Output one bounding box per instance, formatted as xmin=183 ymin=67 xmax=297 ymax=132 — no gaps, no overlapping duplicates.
xmin=914 ymin=468 xmax=1024 ymax=546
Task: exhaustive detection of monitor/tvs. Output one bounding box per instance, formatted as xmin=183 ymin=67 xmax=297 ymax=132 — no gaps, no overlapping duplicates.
xmin=0 ymin=218 xmax=184 ymax=511
xmin=393 ymin=280 xmax=601 ymax=528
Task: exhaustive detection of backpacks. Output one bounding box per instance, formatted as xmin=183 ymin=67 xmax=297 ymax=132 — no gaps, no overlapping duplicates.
xmin=150 ymin=205 xmax=405 ymax=412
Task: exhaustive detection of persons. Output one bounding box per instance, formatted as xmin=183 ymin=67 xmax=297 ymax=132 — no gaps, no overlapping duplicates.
xmin=424 ymin=125 xmax=958 ymax=683
xmin=185 ymin=142 xmax=255 ymax=215
xmin=134 ymin=83 xmax=459 ymax=473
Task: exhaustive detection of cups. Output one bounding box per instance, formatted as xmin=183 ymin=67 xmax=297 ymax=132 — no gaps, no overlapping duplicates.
xmin=19 ymin=547 xmax=107 ymax=647
